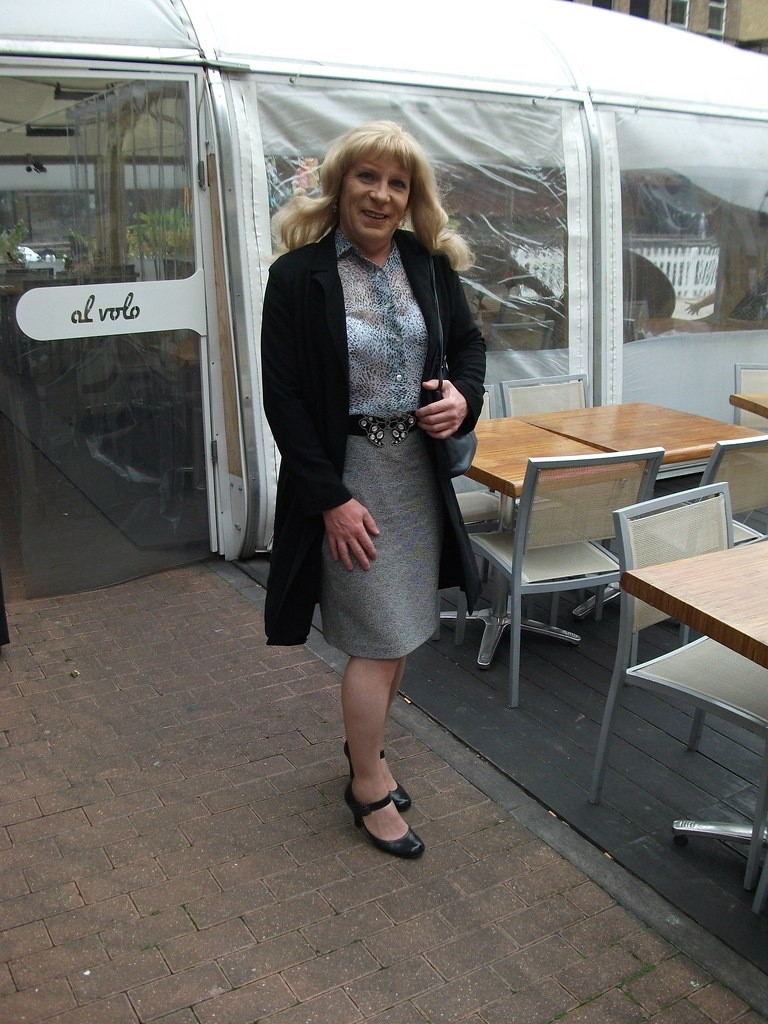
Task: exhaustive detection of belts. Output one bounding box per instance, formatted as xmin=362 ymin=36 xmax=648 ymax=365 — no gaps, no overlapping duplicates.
xmin=347 ymin=410 xmax=420 ymax=447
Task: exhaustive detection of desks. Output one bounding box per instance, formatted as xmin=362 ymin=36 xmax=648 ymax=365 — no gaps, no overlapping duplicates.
xmin=516 ymin=400 xmax=766 ymax=622
xmin=429 ymin=417 xmax=641 ymax=668
xmin=728 ymin=391 xmax=768 ymax=419
xmin=620 ymin=540 xmax=768 ymax=851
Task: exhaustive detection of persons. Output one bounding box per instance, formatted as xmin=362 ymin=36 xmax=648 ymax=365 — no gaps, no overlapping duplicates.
xmin=622 ymin=249 xmax=676 ymax=344
xmin=261 ymin=120 xmax=487 ymax=858
xmin=684 ymin=203 xmax=757 ymax=322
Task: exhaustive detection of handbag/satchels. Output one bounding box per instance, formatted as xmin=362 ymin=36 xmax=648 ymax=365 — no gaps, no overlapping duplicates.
xmin=428 ymin=258 xmax=478 ymax=478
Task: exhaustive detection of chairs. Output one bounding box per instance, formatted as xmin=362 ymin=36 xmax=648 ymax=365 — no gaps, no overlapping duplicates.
xmin=443 ymin=360 xmax=768 ymax=919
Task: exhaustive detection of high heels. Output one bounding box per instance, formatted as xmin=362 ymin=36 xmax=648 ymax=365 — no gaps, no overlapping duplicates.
xmin=342 ymin=777 xmax=425 ymax=858
xmin=344 ymin=739 xmax=412 ymax=812
